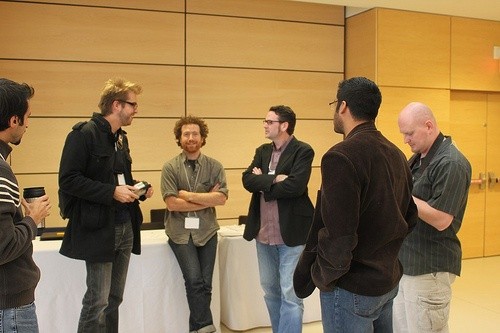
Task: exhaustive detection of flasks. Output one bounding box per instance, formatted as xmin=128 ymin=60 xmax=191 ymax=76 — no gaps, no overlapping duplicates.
xmin=129 ymin=180 xmax=152 ymax=198
xmin=22 ymin=185 xmax=46 ymax=228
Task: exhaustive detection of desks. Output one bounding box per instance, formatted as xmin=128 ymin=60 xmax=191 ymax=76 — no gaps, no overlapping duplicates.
xmin=34 ymin=228 xmax=221 ymax=333
xmin=217 ymin=224 xmax=323 ymax=331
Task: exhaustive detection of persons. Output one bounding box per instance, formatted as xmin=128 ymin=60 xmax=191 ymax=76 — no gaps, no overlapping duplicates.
xmin=0 ymin=78 xmax=51 ymax=333
xmin=242 ymin=105 xmax=316 ymax=333
xmin=57 ymin=77 xmax=155 ymax=333
xmin=392 ymin=102 xmax=473 ymax=333
xmin=293 ymin=77 xmax=418 ymax=333
xmin=160 ymin=116 xmax=230 ymax=333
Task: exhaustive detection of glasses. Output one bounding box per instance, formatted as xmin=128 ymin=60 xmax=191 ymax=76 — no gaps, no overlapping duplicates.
xmin=329 ymin=99 xmax=348 ymax=109
xmin=112 ymin=99 xmax=137 ymax=108
xmin=263 ymin=119 xmax=284 ymax=125
